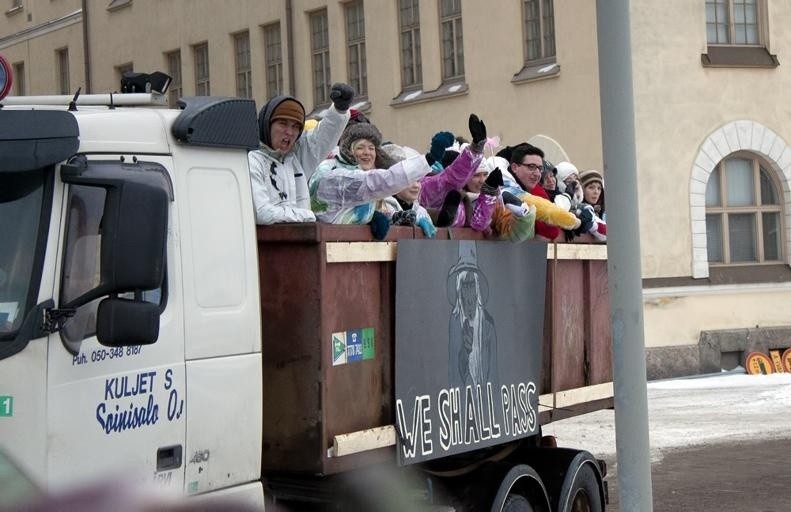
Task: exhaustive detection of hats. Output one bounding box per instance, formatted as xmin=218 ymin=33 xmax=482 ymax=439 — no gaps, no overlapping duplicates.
xmin=542 ymin=159 xmax=558 ymax=181
xmin=444 ymin=240 xmax=490 ymax=309
xmin=580 ymin=169 xmax=604 ymax=188
xmin=554 ymin=161 xmax=581 ymax=183
xmin=271 ymin=99 xmax=306 ymax=127
xmin=461 ymin=142 xmax=491 ymax=176
xmin=344 ymin=108 xmax=372 ymax=130
xmin=340 ymin=122 xmax=397 ymax=170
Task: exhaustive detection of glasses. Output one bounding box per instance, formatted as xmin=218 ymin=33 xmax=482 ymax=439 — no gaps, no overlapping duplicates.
xmin=522 ymin=162 xmax=546 ymax=172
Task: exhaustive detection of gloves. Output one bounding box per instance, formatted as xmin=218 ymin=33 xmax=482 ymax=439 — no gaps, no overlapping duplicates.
xmin=419 ymin=218 xmax=438 ymax=239
xmin=393 ymin=210 xmax=418 ymax=226
xmin=371 ymin=211 xmax=390 ymax=241
xmin=439 ymin=190 xmax=461 ymax=228
xmin=429 ymin=130 xmax=455 ymax=160
xmin=481 ymin=167 xmax=505 ymax=195
xmin=502 ymin=190 xmax=529 ymax=216
xmin=331 ymin=82 xmax=355 ymax=112
xmin=577 ymin=205 xmax=595 ymax=236
xmin=468 ymin=114 xmax=487 ymax=154
xmin=565 ymin=181 xmax=578 ymax=200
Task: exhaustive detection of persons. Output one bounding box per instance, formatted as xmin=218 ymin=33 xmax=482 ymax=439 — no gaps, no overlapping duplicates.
xmin=300 ymin=108 xmax=606 ymax=243
xmin=248 ymin=83 xmax=356 ymax=226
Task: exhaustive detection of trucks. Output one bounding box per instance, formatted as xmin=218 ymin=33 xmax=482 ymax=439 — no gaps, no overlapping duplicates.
xmin=0 ymin=93 xmax=615 ymax=512
